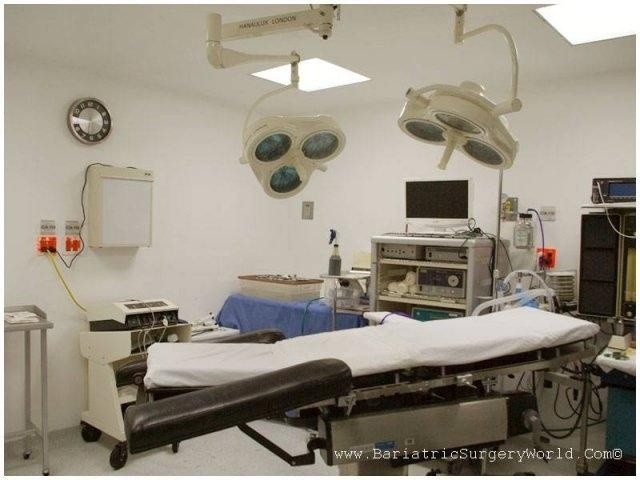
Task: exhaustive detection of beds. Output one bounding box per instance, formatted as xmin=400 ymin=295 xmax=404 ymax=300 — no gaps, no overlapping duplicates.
xmin=115 ymin=305 xmax=599 ymax=474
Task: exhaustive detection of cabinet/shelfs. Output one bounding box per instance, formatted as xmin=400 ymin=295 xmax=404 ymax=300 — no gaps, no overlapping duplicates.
xmin=372 ymin=235 xmax=507 ymax=319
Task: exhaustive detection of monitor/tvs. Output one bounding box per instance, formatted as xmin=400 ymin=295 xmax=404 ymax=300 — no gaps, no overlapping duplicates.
xmin=403 ymin=176 xmax=472 ymax=236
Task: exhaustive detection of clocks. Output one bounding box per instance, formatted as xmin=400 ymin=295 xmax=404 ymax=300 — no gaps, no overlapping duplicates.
xmin=69 ymin=96 xmax=113 ymax=146
